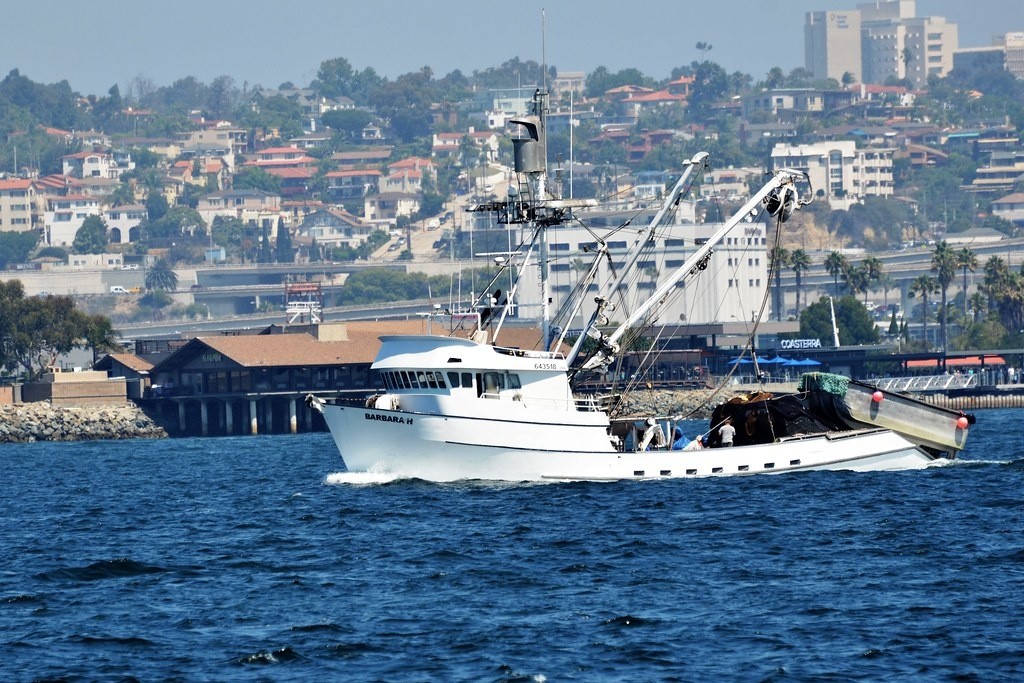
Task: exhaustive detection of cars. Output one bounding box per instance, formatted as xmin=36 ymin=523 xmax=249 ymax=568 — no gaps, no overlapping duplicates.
xmin=427 ymin=210 xmax=455 ymax=231
xmin=36 ymin=290 xmax=55 ymax=298
xmin=471 ymin=195 xmax=480 ymax=203
xmin=899 ymin=240 xmax=936 ymax=249
xmin=389 ymin=228 xmax=402 ymax=237
xmin=483 ymin=184 xmax=496 ymax=192
xmin=128 ymin=286 xmax=145 ymax=293
xmin=861 ymin=301 xmax=904 ymax=319
xmin=432 ymin=240 xmax=444 ymax=249
xmin=388 ymin=243 xmax=400 ymax=252
xmin=397 ymin=237 xmax=406 ymax=245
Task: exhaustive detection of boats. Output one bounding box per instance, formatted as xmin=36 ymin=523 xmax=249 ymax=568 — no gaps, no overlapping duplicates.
xmin=798 ymin=371 xmax=976 ymax=456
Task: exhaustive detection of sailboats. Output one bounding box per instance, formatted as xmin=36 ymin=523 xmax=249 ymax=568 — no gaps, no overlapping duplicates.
xmin=303 ymin=3 xmax=977 ymax=487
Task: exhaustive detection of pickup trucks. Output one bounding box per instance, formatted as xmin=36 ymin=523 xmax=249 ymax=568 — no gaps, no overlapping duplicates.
xmin=120 ymin=264 xmax=139 ymax=271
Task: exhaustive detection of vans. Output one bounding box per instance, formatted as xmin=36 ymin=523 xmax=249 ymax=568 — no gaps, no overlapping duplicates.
xmin=110 ymin=286 xmax=131 ymax=295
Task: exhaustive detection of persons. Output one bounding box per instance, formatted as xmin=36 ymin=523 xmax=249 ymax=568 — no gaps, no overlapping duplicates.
xmin=717 ymin=420 xmax=736 ymax=448
xmin=944 ymin=368 xmax=973 ymax=385
xmin=1007 ymin=365 xmax=1014 ymax=385
